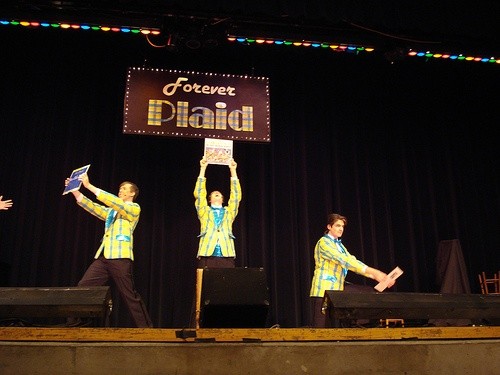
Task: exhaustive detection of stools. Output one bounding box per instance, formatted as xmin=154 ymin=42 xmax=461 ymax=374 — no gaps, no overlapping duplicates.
xmin=380 ymin=319 xmax=404 ymax=328
xmin=479 ymin=271 xmax=500 ymax=294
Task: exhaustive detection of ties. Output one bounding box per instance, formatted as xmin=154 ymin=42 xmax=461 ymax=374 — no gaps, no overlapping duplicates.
xmin=105 ymin=208 xmax=114 ymax=228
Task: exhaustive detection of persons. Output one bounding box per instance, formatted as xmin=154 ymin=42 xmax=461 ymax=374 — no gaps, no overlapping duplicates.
xmin=189 ymin=156 xmax=242 ymax=327
xmin=61 ymin=164 xmax=155 ymax=329
xmin=0 ymin=195 xmax=13 ymax=211
xmin=307 ymin=213 xmax=396 ymax=329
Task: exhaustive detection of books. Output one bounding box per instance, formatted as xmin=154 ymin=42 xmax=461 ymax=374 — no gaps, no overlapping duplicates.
xmin=373 ymin=266 xmax=403 ymax=293
xmin=62 ymin=164 xmax=91 ymax=195
xmin=203 ymin=137 xmax=233 ymax=165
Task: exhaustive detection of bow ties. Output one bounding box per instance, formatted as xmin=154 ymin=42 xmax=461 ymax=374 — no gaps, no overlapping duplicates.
xmin=213 ymin=208 xmax=225 ymax=211
xmin=334 ymin=239 xmax=342 ymax=245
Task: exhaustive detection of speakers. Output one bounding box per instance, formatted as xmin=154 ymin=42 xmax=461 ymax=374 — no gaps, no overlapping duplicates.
xmin=190 ymin=266 xmax=272 ymax=328
xmin=0 ymin=287 xmax=113 ymax=327
xmin=322 ymin=290 xmax=500 ymax=328
